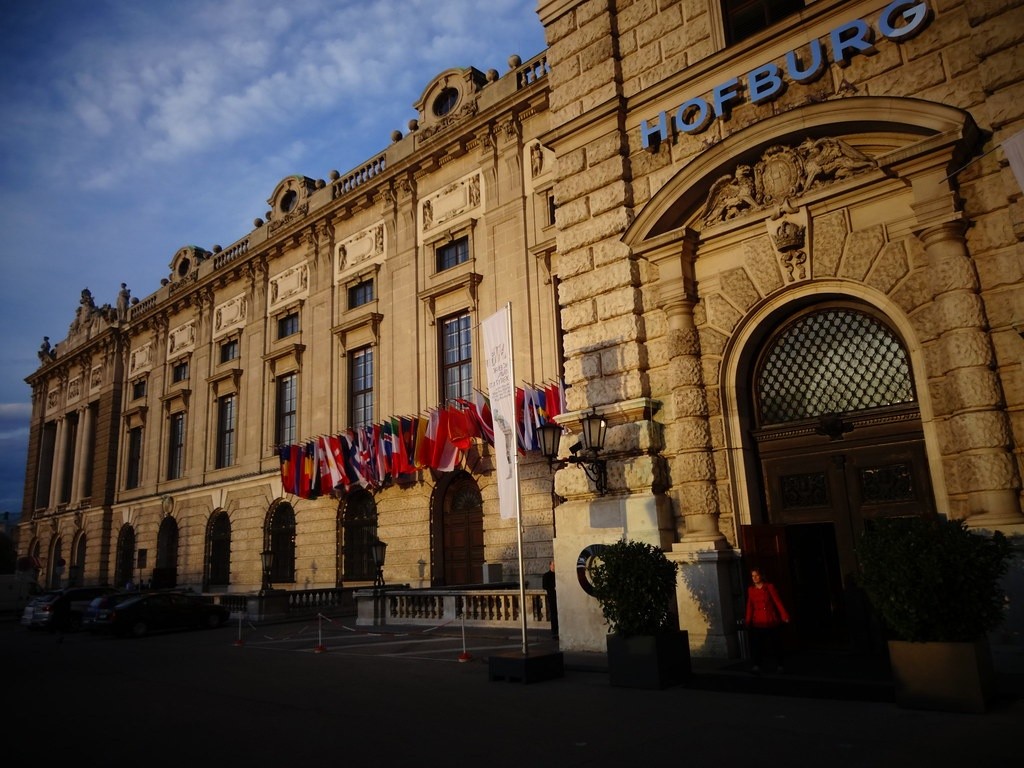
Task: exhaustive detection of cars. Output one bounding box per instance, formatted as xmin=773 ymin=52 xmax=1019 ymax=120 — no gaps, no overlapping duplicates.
xmin=94 ymin=591 xmax=230 ymax=639
xmin=21 ymin=585 xmax=142 ymax=633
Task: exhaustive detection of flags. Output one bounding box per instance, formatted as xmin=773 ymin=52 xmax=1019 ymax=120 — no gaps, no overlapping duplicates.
xmin=31 ymin=542 xmax=40 ymax=573
xmin=281 ymin=375 xmax=569 ymax=499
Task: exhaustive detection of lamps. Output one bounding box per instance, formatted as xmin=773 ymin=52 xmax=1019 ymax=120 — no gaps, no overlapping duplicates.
xmin=259 ymin=543 xmax=275 ymax=571
xmin=369 ymin=535 xmax=388 ymax=567
xmin=568 ymin=405 xmax=608 ymax=497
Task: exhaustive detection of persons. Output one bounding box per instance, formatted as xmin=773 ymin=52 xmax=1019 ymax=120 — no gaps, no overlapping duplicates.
xmin=542 ymin=561 xmax=559 ymax=639
xmin=126 ymin=579 xmax=154 ymax=594
xmin=118 ymin=283 xmax=129 ymax=320
xmin=744 ymin=567 xmax=790 ymax=671
xmin=39 ymin=336 xmax=51 ymax=354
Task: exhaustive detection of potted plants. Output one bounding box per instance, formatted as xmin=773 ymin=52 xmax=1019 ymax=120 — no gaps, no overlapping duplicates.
xmin=594 ymin=539 xmax=694 ymax=692
xmin=857 ymin=512 xmax=1018 ymax=713
